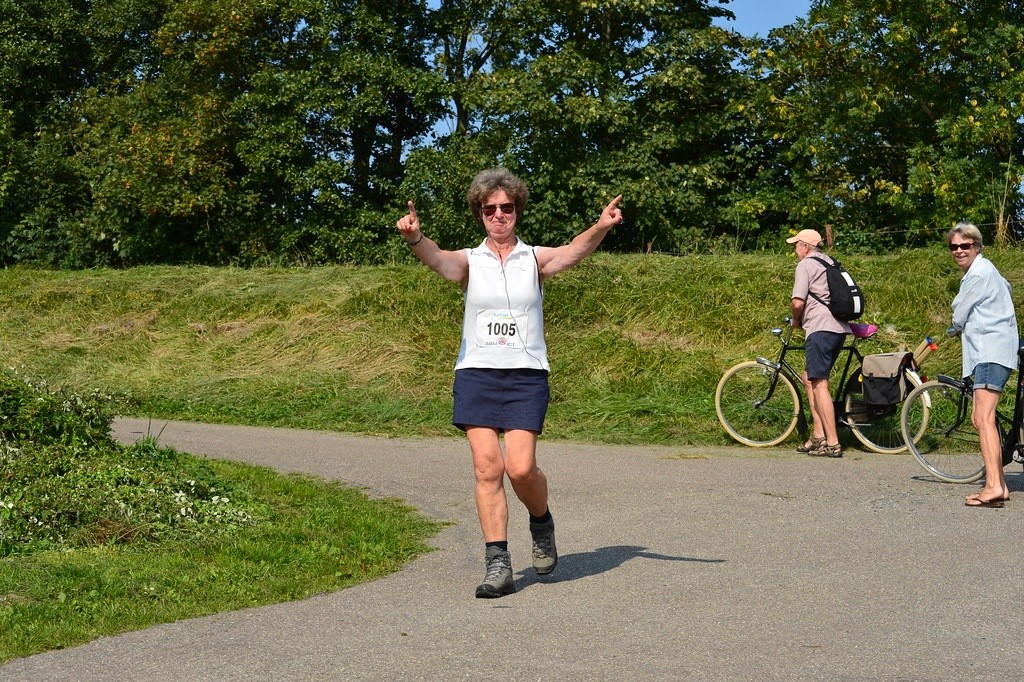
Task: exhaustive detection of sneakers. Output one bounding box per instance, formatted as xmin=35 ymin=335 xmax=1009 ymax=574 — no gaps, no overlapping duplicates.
xmin=528 ymin=516 xmax=558 ymax=574
xmin=474 ymin=546 xmax=515 ymax=599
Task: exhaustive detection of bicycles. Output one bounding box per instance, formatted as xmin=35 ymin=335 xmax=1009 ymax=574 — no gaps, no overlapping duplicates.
xmin=900 ymin=331 xmax=1024 ymax=485
xmin=715 ymin=317 xmax=932 ymax=454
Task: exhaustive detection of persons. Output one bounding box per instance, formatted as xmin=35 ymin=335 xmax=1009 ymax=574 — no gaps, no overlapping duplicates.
xmin=786 ymin=229 xmax=853 ymax=458
xmin=396 ymin=165 xmax=622 ymax=598
xmin=948 ymin=223 xmax=1019 ymax=506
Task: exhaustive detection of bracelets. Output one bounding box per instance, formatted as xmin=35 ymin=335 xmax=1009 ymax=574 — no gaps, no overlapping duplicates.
xmin=408 ymin=233 xmax=423 ymax=246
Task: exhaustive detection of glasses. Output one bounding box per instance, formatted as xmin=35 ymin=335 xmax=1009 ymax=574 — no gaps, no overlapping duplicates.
xmin=950 ymin=242 xmax=976 ymax=251
xmin=477 ymin=202 xmax=516 ymax=216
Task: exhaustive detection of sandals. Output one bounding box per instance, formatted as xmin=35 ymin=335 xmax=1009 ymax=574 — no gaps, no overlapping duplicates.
xmin=808 ymin=443 xmax=843 ymax=458
xmin=797 ymin=435 xmax=827 ymax=453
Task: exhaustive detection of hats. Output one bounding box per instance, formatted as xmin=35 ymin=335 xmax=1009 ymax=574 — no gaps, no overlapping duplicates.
xmin=786 ymin=229 xmax=822 ymax=247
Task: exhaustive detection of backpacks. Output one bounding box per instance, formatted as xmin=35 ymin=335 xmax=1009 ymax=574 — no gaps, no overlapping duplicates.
xmin=805 ymin=255 xmax=865 ymax=321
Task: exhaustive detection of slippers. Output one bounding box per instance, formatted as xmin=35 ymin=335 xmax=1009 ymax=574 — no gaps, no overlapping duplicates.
xmin=964 ymin=492 xmax=1011 ymax=507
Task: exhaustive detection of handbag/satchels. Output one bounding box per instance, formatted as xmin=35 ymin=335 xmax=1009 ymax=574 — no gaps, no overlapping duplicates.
xmin=861 ymin=351 xmax=914 ymax=406
